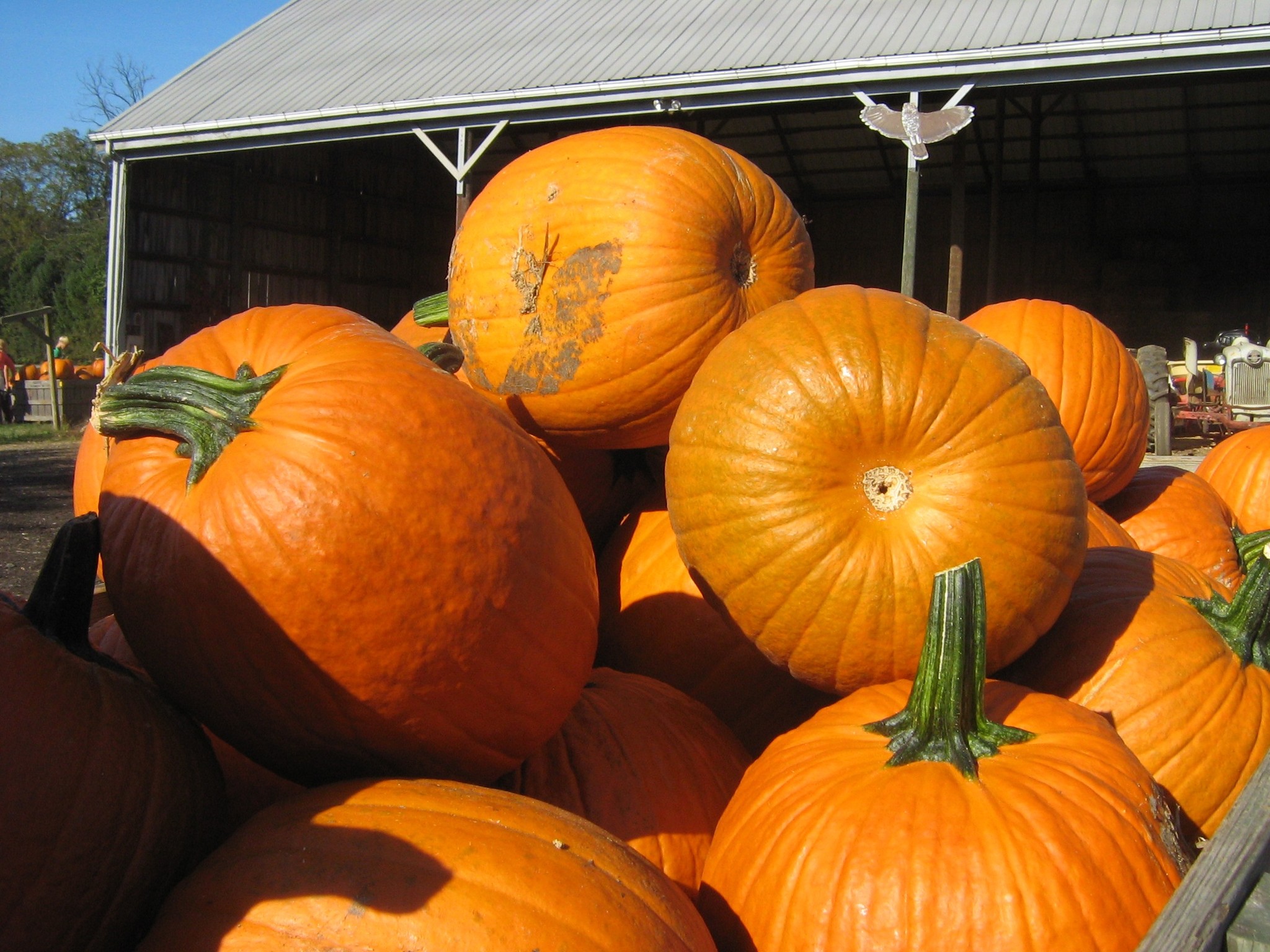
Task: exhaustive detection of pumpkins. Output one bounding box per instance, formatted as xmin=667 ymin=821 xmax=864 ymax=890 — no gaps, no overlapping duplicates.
xmin=0 ymin=127 xmax=1270 ymax=952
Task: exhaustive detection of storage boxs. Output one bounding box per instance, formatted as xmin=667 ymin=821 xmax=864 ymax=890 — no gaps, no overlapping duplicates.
xmin=12 ymin=379 xmax=101 ymax=423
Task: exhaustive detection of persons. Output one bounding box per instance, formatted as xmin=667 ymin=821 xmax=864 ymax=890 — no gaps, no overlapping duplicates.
xmin=52 ymin=336 xmax=71 ymax=359
xmin=0 ymin=339 xmax=17 ymax=425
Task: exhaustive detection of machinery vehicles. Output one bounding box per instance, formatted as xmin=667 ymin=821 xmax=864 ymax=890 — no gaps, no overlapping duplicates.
xmin=1136 ymin=322 xmax=1270 ymax=456
